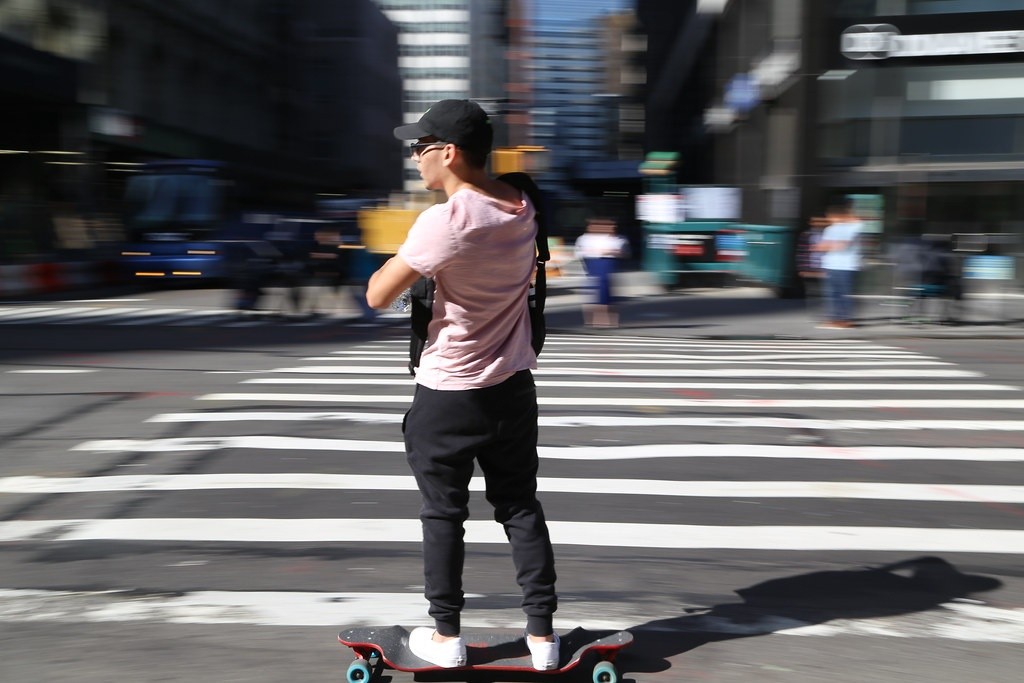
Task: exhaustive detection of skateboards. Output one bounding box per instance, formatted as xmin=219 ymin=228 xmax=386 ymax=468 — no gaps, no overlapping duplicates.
xmin=336 ymin=622 xmax=635 ymax=683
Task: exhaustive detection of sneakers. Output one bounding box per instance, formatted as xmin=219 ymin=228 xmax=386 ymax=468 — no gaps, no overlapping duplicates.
xmin=408 ymin=626 xmax=467 ymax=669
xmin=523 ymin=627 xmax=561 ymax=672
xmin=816 ymin=318 xmax=850 ymax=329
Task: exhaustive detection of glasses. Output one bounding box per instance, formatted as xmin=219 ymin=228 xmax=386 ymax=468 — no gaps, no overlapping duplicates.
xmin=409 ymin=142 xmax=449 ymax=155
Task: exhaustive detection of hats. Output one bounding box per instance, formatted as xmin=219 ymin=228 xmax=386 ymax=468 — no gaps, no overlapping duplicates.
xmin=392 ymin=98 xmax=494 ymax=153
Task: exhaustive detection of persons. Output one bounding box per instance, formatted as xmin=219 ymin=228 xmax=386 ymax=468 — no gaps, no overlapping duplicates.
xmin=364 ymin=99 xmax=568 ymax=674
xmin=573 ymin=213 xmax=628 ymax=305
xmin=812 ymin=204 xmax=866 ymax=329
